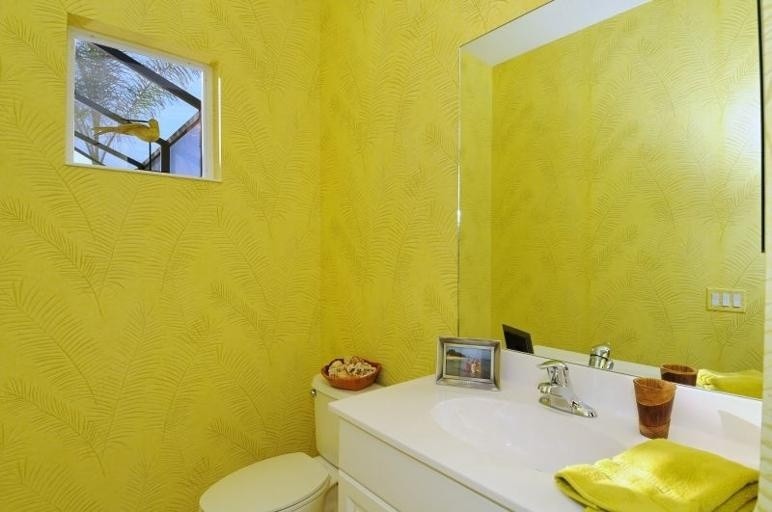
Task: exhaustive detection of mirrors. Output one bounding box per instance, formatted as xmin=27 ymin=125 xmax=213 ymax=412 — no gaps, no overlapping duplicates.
xmin=456 ymin=2 xmax=767 ymax=402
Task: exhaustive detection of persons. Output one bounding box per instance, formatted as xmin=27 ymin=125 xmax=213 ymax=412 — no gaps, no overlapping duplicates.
xmin=475 ymin=361 xmax=481 ymax=373
xmin=470 ymin=360 xmax=476 ymax=374
xmin=465 ymin=356 xmax=472 ymax=371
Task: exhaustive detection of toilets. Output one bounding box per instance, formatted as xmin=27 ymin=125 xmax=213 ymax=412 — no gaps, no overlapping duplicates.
xmin=199 ymin=372 xmax=386 ymax=511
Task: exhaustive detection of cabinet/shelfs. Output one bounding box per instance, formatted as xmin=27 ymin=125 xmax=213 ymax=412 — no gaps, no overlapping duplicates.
xmin=327 ymin=408 xmax=513 ymax=512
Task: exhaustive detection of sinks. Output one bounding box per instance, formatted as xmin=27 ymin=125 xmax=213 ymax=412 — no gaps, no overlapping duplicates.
xmin=430 ymin=396 xmax=611 ymax=472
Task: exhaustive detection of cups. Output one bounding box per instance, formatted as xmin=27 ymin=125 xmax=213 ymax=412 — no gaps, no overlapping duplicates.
xmin=632 ymin=377 xmax=678 ymax=439
xmin=659 ymin=362 xmax=698 ymax=386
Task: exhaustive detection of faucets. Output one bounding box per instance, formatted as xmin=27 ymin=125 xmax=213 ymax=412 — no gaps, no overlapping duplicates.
xmin=589 ymin=344 xmax=614 ymax=371
xmin=537 ymin=359 xmax=598 ymax=418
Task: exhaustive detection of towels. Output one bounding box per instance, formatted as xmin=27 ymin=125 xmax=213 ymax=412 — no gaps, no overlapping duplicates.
xmin=552 ymin=438 xmax=760 ymax=511
xmin=696 ymin=368 xmax=762 ymax=398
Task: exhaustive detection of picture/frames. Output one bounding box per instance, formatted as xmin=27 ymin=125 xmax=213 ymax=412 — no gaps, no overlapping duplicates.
xmin=501 ymin=323 xmax=534 ymax=356
xmin=436 ymin=336 xmax=501 ymax=392
